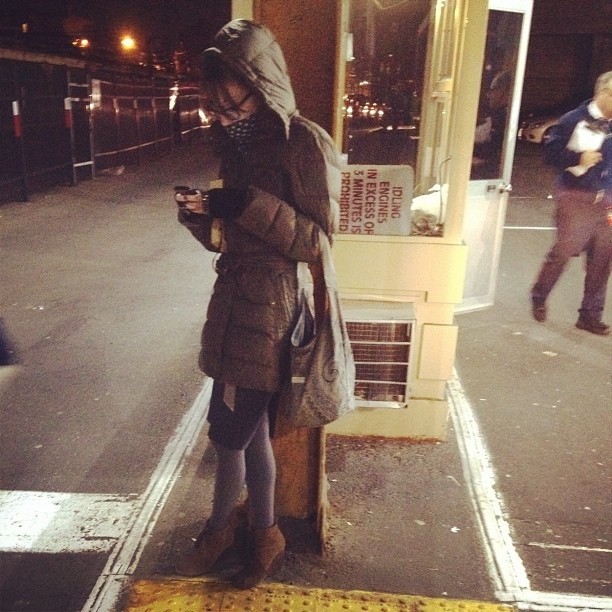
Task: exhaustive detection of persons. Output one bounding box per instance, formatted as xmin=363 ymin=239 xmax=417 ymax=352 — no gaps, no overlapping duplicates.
xmin=472 ymin=69 xmax=516 ymax=180
xmin=532 ymin=73 xmax=612 ymax=337
xmin=173 ymin=18 xmax=342 ymax=589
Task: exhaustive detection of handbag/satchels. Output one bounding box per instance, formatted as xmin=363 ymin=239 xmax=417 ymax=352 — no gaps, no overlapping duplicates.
xmin=271 ymin=228 xmax=355 ymax=435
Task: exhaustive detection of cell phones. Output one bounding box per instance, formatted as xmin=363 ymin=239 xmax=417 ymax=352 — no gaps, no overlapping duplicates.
xmin=173 ymin=186 xmax=189 ymax=206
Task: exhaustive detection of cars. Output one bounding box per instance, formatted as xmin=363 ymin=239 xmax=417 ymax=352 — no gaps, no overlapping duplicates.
xmin=517 ymin=96 xmax=595 ymax=146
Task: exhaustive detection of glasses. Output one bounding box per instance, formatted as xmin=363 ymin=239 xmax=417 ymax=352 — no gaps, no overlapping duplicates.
xmin=206 ymin=91 xmax=254 ymax=124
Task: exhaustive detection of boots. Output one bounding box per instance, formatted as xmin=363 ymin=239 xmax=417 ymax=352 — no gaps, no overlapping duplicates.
xmin=175 ymin=519 xmax=234 ymax=576
xmin=233 ymin=524 xmax=285 ymax=589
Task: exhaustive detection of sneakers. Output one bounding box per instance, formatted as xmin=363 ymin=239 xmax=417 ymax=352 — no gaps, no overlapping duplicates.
xmin=576 ymin=317 xmax=611 ymax=335
xmin=532 ymin=295 xmax=547 ymax=321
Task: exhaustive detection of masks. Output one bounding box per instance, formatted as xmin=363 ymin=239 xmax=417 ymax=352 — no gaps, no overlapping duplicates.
xmin=218 ymin=113 xmax=258 ymax=142
